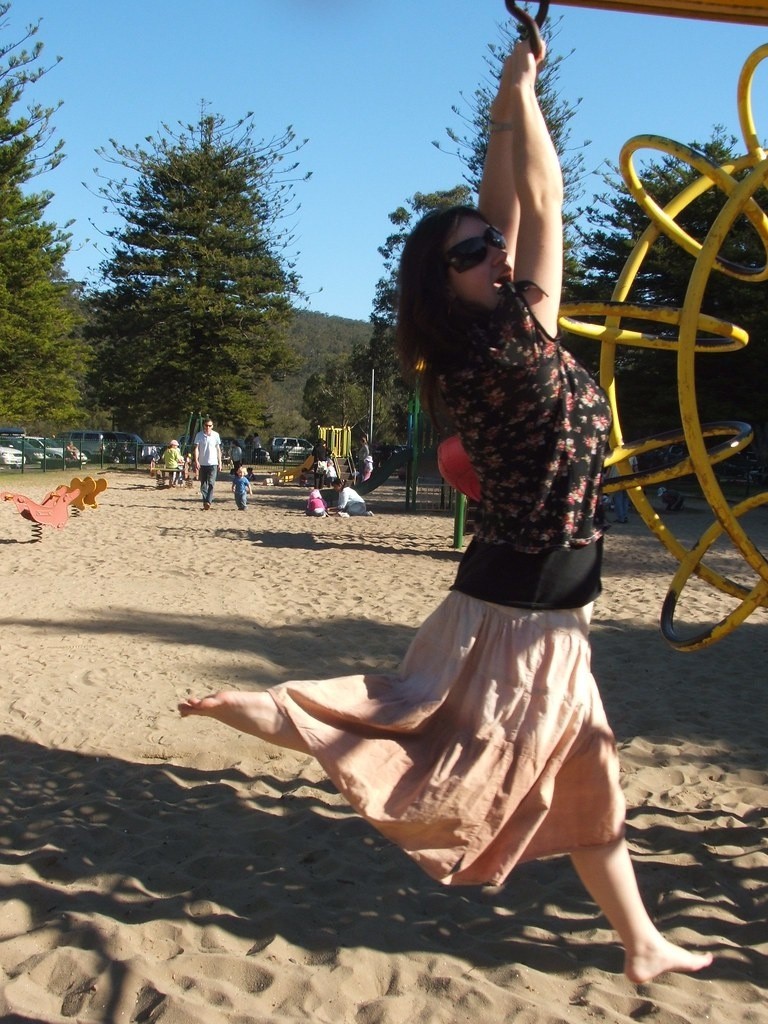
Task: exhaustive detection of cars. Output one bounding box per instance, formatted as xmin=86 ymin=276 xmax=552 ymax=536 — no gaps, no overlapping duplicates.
xmin=0 ymin=446 xmax=27 ymax=469
xmin=15 ymin=436 xmax=88 ymax=461
xmin=56 ymin=430 xmax=151 ymax=463
xmin=220 ymin=436 xmax=269 ymax=464
xmin=178 ymin=434 xmax=193 ymax=452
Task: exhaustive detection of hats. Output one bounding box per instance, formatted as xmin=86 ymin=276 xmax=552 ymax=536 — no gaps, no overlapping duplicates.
xmin=310 ymin=488 xmax=322 ymax=499
xmin=364 ymin=456 xmax=373 ymax=464
xmin=169 ymin=440 xmax=179 ymax=446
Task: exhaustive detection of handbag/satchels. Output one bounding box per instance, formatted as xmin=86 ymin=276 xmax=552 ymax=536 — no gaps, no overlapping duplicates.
xmin=316 ymin=460 xmax=327 ymax=475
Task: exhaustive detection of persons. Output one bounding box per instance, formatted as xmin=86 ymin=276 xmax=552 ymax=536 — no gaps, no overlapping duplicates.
xmin=307 ymin=488 xmax=329 ymax=518
xmin=231 ymin=469 xmax=252 ymax=510
xmin=358 ymin=436 xmax=374 ymax=482
xmin=64 ymin=440 xmax=80 ymax=461
xmin=245 ymin=468 xmax=256 ymax=481
xmin=161 ymin=439 xmax=192 ymax=484
xmin=192 ymin=419 xmax=223 ymax=510
xmin=330 ymin=479 xmax=375 ymax=518
xmin=229 ymin=440 xmax=242 ymax=473
xmin=311 ymin=438 xmax=337 ymax=489
xmin=181 ymin=39 xmax=717 ymax=987
xmin=245 ymin=432 xmax=274 ymax=464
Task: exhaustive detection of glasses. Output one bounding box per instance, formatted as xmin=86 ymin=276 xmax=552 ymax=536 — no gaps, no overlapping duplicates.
xmin=204 ymin=424 xmax=213 ymax=427
xmin=444 ymin=226 xmax=507 ymax=275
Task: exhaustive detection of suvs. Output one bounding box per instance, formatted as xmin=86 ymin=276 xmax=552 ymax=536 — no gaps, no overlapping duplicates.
xmin=0 ymin=426 xmax=56 ymax=464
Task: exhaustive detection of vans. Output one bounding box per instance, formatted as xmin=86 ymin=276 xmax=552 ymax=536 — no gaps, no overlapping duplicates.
xmin=270 ymin=436 xmax=315 ymax=463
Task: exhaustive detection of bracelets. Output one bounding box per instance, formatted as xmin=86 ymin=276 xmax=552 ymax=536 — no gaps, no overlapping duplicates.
xmin=484 ymin=120 xmax=515 ymax=132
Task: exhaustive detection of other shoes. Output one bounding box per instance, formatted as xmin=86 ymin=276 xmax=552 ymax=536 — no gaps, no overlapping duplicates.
xmin=204 ymin=502 xmax=210 ymax=510
